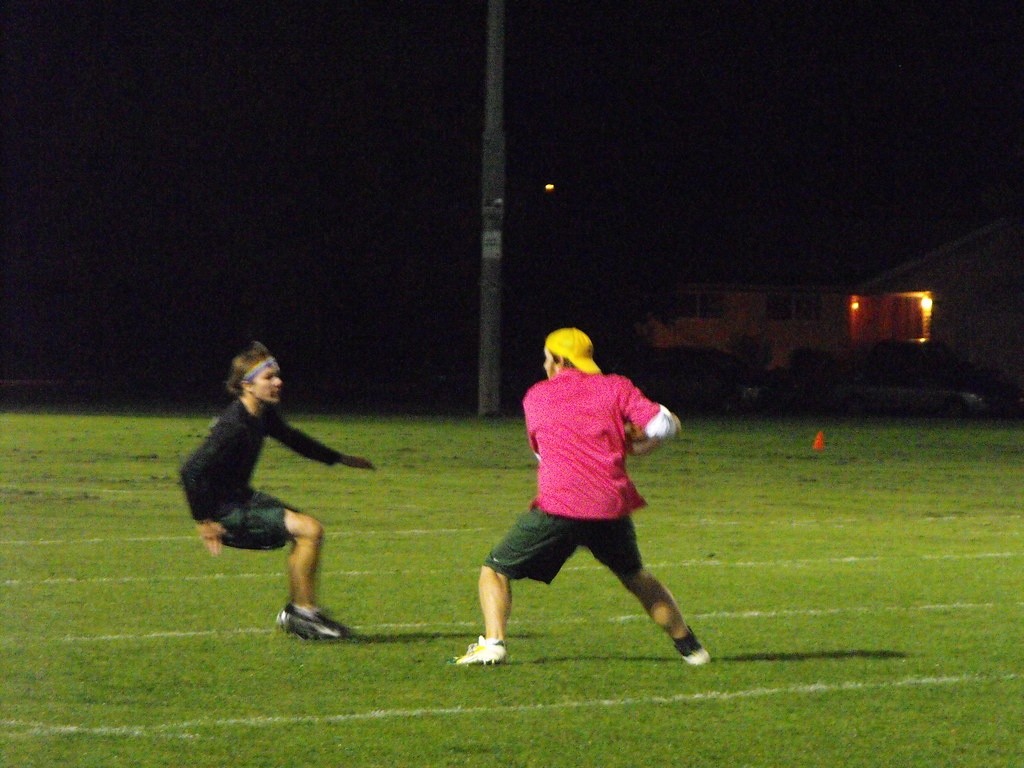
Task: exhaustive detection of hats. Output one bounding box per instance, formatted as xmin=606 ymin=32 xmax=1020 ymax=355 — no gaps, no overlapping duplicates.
xmin=546 ymin=326 xmax=603 ymax=375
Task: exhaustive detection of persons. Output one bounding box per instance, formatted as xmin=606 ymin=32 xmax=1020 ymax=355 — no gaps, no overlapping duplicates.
xmin=178 ymin=342 xmax=375 ymax=640
xmin=453 ymin=328 xmax=712 ymax=665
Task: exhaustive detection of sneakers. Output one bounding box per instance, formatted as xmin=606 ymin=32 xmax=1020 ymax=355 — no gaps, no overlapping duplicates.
xmin=275 ymin=602 xmax=350 ymax=644
xmin=455 ymin=636 xmax=508 ymax=666
xmin=685 ymin=645 xmax=712 ymax=668
